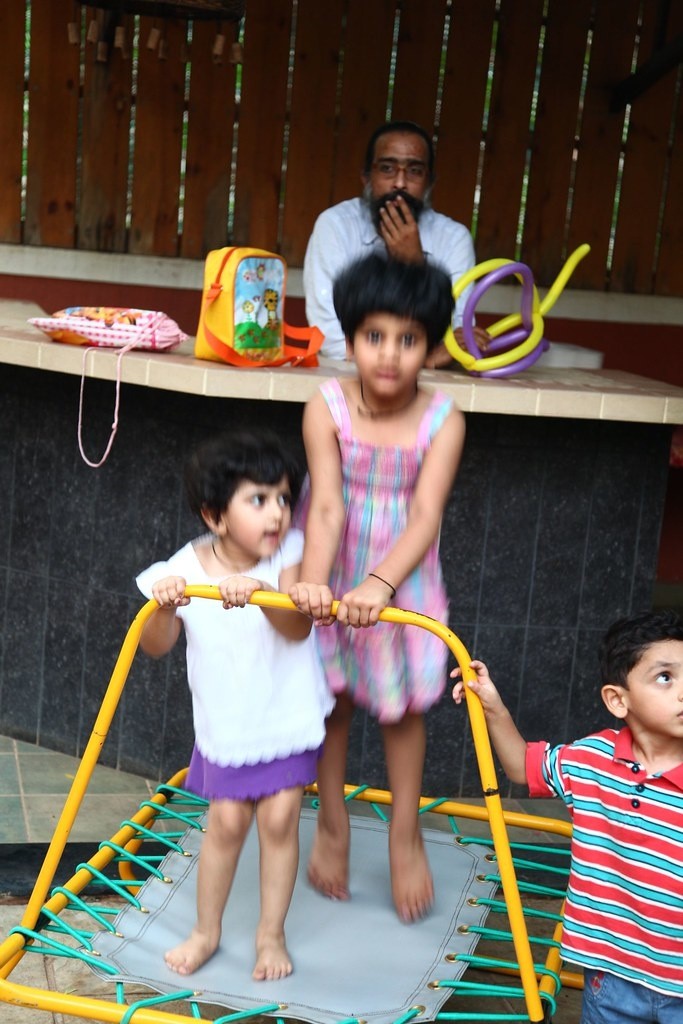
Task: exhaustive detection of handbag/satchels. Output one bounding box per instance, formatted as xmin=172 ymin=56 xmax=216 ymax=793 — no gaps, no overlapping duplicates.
xmin=27 ymin=305 xmax=190 ymax=352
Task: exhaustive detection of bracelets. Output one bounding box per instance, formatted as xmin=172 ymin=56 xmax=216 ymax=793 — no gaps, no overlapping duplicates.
xmin=369 ymin=573 xmax=396 ymax=599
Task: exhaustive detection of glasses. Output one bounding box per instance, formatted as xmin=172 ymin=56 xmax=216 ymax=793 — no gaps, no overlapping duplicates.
xmin=371 ymin=161 xmax=430 ymax=184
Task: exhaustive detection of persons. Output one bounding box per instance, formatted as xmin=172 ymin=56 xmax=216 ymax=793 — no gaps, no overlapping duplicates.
xmin=138 ymin=431 xmax=335 ymax=982
xmin=450 ymin=612 xmax=683 ymax=1024
xmin=304 ymin=120 xmax=492 ymax=370
xmin=291 ymin=254 xmax=465 ymax=922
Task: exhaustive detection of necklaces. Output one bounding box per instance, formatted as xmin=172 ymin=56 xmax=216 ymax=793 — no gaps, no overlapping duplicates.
xmin=211 ymin=536 xmax=260 ymax=570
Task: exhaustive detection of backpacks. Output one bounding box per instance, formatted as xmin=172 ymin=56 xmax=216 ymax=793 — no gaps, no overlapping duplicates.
xmin=194 ymin=245 xmax=325 ymax=367
